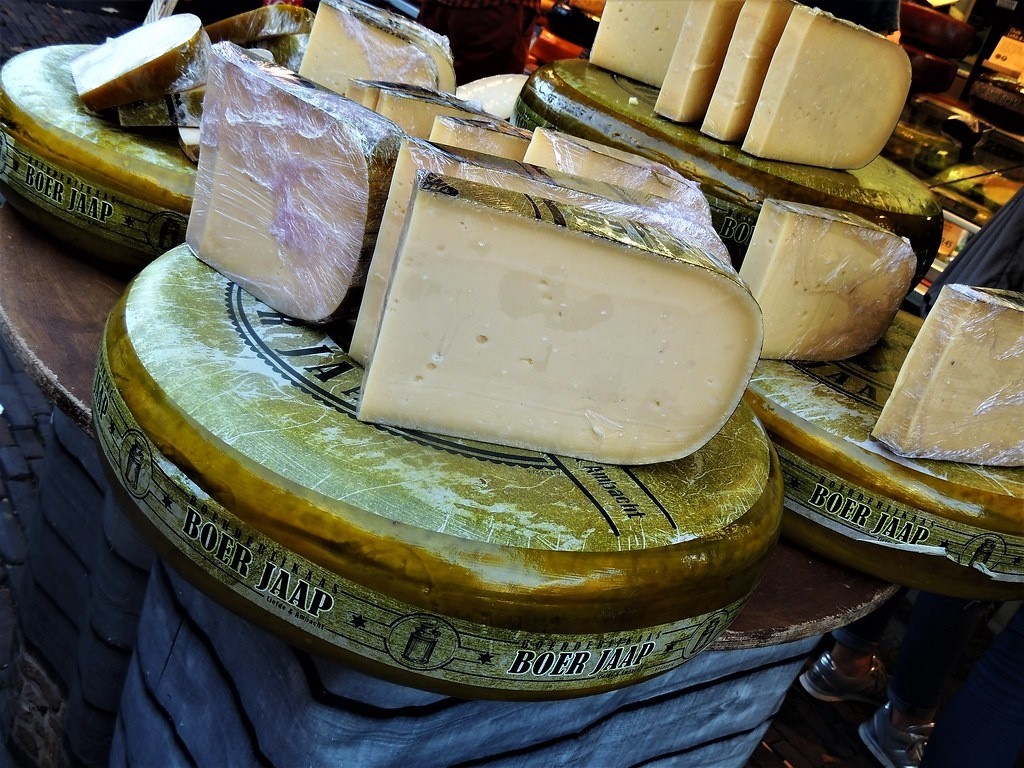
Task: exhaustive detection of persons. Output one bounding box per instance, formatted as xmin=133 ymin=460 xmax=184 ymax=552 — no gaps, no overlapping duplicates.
xmin=801 ymin=184 xmax=1024 ymax=768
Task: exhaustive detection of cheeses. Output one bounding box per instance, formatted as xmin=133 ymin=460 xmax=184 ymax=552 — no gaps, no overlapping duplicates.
xmin=1 ymin=0 xmax=1024 ymax=702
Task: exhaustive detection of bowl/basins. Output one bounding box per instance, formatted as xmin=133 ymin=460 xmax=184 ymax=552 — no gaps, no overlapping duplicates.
xmin=880 ymin=120 xmax=962 ymax=180
xmin=879 ymin=0 xmax=973 ymax=97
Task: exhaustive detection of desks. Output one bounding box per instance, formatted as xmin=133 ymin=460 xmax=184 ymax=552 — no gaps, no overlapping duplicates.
xmin=0 ymin=191 xmax=904 ymax=768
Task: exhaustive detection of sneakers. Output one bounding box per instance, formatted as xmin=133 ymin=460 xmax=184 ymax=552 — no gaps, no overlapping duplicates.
xmin=798 ymin=649 xmax=896 ymax=701
xmin=858 ymin=700 xmax=936 ymax=768
